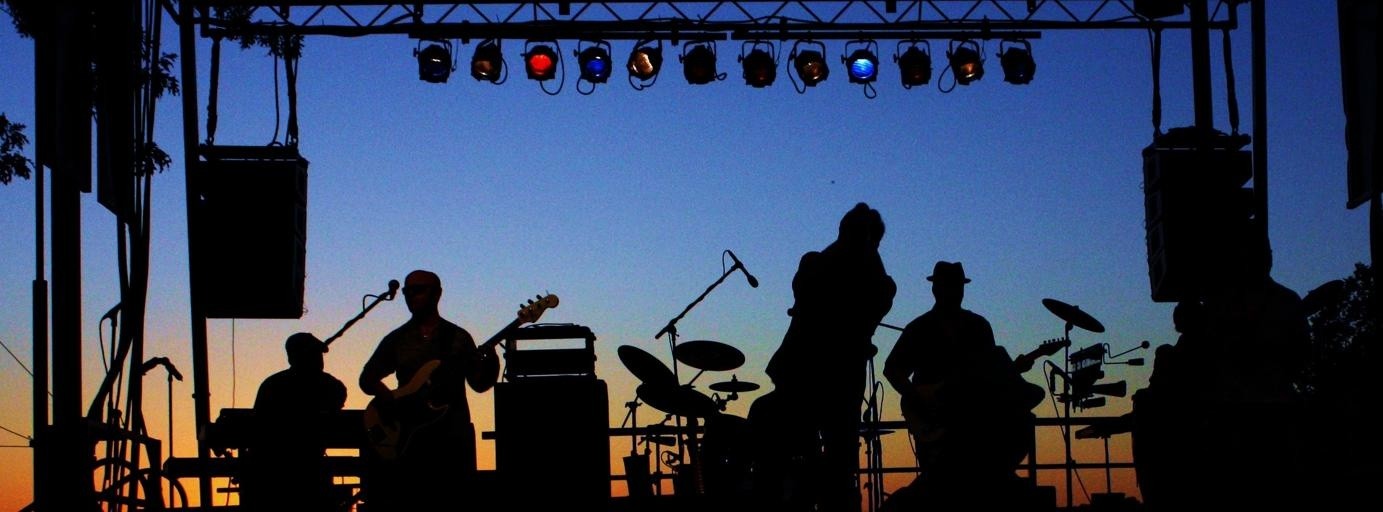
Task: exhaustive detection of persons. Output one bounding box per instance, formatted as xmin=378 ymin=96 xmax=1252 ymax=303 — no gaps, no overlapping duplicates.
xmin=239 ymin=329 xmax=352 ymax=511
xmin=764 ymin=198 xmax=898 ymax=511
xmin=883 ymin=261 xmax=1042 ymax=483
xmin=357 ymin=269 xmax=501 ymax=511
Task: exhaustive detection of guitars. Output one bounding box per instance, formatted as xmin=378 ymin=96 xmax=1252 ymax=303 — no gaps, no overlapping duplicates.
xmin=364 ymin=296 xmax=557 ymax=462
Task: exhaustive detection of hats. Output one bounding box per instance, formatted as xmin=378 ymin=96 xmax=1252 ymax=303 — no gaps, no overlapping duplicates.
xmin=404 ymin=272 xmax=440 ymax=288
xmin=926 ymin=261 xmax=971 ymax=283
xmin=285 ymin=332 xmax=329 ymax=353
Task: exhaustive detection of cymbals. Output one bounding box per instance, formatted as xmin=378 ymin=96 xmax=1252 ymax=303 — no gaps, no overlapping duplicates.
xmin=637 ymin=385 xmax=716 ymax=413
xmin=619 ymin=346 xmax=677 ymax=384
xmin=1042 ymin=298 xmax=1103 ymax=333
xmin=674 ymin=341 xmax=745 ymax=370
xmin=712 ymin=382 xmax=757 ymax=390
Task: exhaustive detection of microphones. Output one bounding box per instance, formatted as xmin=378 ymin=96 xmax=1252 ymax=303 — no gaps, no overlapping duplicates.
xmin=388 ymin=279 xmax=400 ymax=301
xmin=725 ymin=249 xmax=759 ymax=287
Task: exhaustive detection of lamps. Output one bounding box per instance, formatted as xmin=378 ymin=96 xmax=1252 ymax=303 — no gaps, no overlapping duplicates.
xmin=946 ymin=46 xmax=988 ymax=84
xmin=521 ymin=37 xmax=564 ymax=85
xmin=841 ymin=48 xmax=884 ymax=84
xmin=409 ymin=38 xmax=465 ymax=84
xmin=798 ymin=48 xmax=831 ymax=83
xmin=892 ymin=45 xmax=935 ymax=84
xmin=469 ymin=36 xmax=513 ymax=86
xmin=625 ymin=40 xmax=665 ymax=81
xmin=678 ymin=45 xmax=724 ymax=83
xmin=574 ymin=39 xmax=617 ymax=84
xmin=995 ymin=46 xmax=1034 ymax=85
xmin=737 ymin=46 xmax=781 ymax=85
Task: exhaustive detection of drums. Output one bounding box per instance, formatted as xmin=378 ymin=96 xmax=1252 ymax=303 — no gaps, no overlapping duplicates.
xmin=699 ymin=414 xmax=751 ymax=466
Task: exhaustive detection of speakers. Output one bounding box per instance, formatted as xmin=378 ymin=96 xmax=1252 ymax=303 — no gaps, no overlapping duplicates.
xmin=494 ymin=376 xmax=609 ymax=493
xmin=1144 ymin=132 xmax=1259 ymax=303
xmin=185 ymin=160 xmax=307 ymax=319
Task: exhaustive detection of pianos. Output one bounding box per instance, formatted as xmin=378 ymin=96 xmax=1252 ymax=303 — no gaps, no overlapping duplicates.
xmin=201 ymin=409 xmax=374 ymax=452
